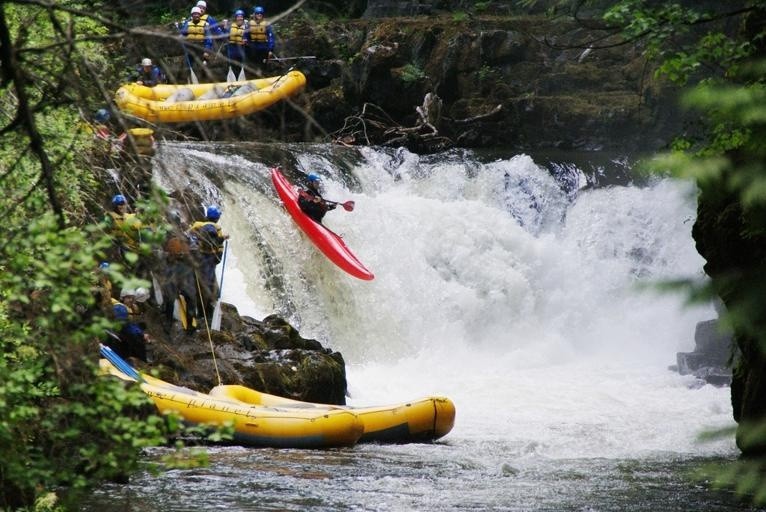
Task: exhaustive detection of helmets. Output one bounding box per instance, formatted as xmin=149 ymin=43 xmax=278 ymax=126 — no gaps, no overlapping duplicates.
xmin=140 ymin=58 xmax=152 ymax=66
xmin=307 ymin=175 xmax=320 ymax=181
xmin=120 ymin=286 xmax=149 ymax=303
xmin=235 ymin=6 xmax=264 ymax=16
xmin=206 ymin=205 xmax=220 ymax=218
xmin=191 ymin=1 xmax=207 ymax=15
xmin=112 ymin=194 xmax=127 ymax=204
xmin=114 ymin=304 xmax=128 ymax=319
xmin=95 ymin=109 xmax=112 ymax=122
xmin=167 ymin=211 xmax=180 ymax=225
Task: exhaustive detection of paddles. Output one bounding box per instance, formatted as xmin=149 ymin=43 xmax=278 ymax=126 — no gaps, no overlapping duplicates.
xmin=298 ymin=188 xmax=354 ymax=211
xmin=211 ymin=241 xmax=230 ymax=330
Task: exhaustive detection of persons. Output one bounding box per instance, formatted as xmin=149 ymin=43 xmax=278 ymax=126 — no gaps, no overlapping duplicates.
xmin=178 ymin=7 xmax=210 ymax=84
xmin=298 ymin=171 xmax=336 ymax=225
xmin=244 ymin=6 xmax=275 ymax=83
xmin=129 ymin=58 xmax=167 ymax=88
xmin=222 ymin=8 xmax=248 ymax=84
xmin=190 ymin=0 xmax=227 ymax=50
xmin=113 ymin=121 xmax=153 ymax=172
xmin=98 ymin=195 xmax=229 ymax=366
xmin=89 ymin=109 xmax=113 ymax=157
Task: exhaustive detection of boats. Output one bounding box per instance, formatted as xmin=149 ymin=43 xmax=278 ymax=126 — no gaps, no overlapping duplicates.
xmin=94 ymin=357 xmax=456 ymax=448
xmin=270 ymin=165 xmax=375 ymax=279
xmin=114 ymin=70 xmax=308 ymax=124
